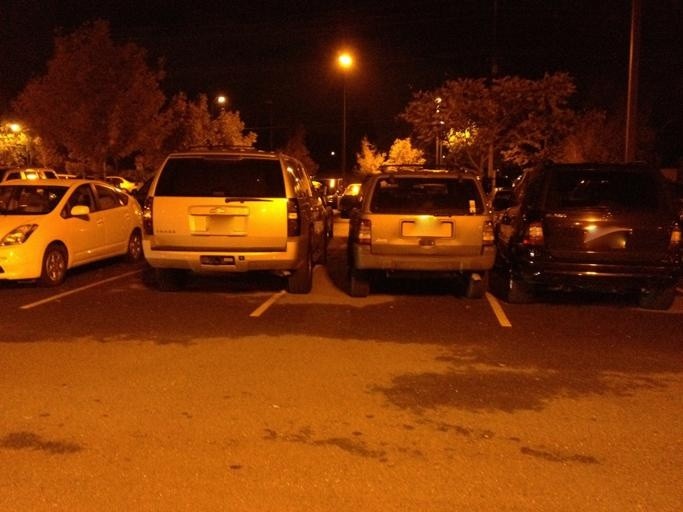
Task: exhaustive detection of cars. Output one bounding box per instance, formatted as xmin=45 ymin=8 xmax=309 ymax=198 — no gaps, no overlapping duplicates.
xmin=491 ymin=162 xmax=682 ymax=311
xmin=105 ymin=177 xmax=156 ymax=224
xmin=0 ymin=179 xmax=144 ymax=285
xmin=311 ymin=176 xmax=363 ymax=213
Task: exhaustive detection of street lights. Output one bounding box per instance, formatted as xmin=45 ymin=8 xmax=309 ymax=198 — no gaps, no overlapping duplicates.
xmin=207 ymin=95 xmax=226 ymax=118
xmin=334 ymin=47 xmax=353 ymax=186
xmin=431 ymin=94 xmax=444 ymax=164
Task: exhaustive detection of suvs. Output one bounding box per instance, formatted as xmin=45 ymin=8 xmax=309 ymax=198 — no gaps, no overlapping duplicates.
xmin=2 ymin=166 xmax=60 ymax=183
xmin=137 ymin=143 xmax=332 ymax=294
xmin=337 ymin=168 xmax=496 ymax=299
